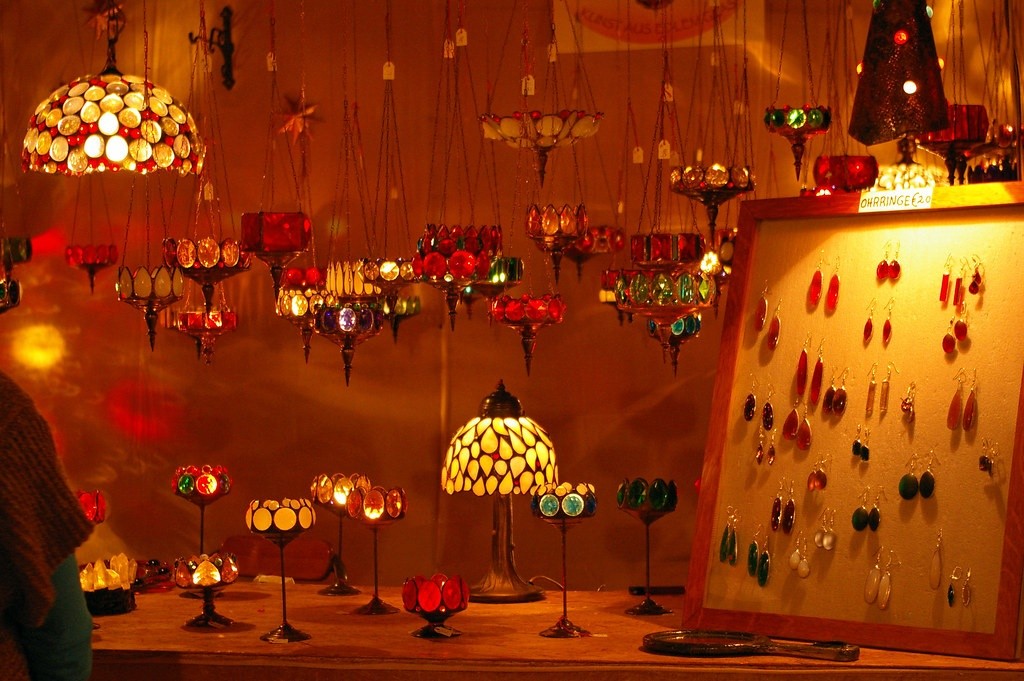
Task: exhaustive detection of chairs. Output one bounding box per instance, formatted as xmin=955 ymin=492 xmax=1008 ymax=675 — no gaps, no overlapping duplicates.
xmin=208 ymin=536 xmax=350 ymax=584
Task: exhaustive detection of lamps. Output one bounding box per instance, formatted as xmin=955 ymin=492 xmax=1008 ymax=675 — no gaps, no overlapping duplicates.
xmin=0 ymin=1 xmax=1024 ymax=639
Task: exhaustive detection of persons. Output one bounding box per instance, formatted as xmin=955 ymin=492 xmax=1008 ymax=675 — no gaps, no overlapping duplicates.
xmin=0 ymin=372 xmax=99 ymax=681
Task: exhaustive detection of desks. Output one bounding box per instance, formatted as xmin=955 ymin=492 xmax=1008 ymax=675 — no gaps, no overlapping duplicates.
xmin=91 ymin=583 xmax=1024 ymax=681
